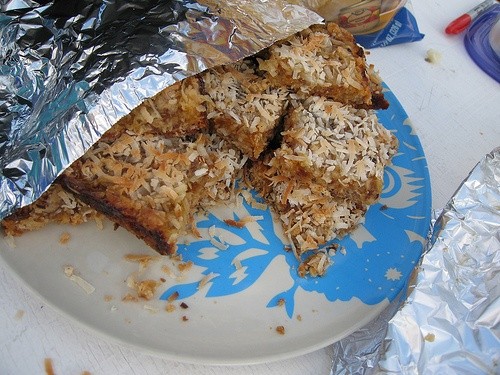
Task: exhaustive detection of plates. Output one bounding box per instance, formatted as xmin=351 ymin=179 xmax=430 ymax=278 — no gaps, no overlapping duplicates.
xmin=0 ymin=71 xmax=431 ymax=365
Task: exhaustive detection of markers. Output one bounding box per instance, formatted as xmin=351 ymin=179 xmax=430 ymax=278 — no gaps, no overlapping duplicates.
xmin=445 ymin=0 xmax=498 ymax=34
